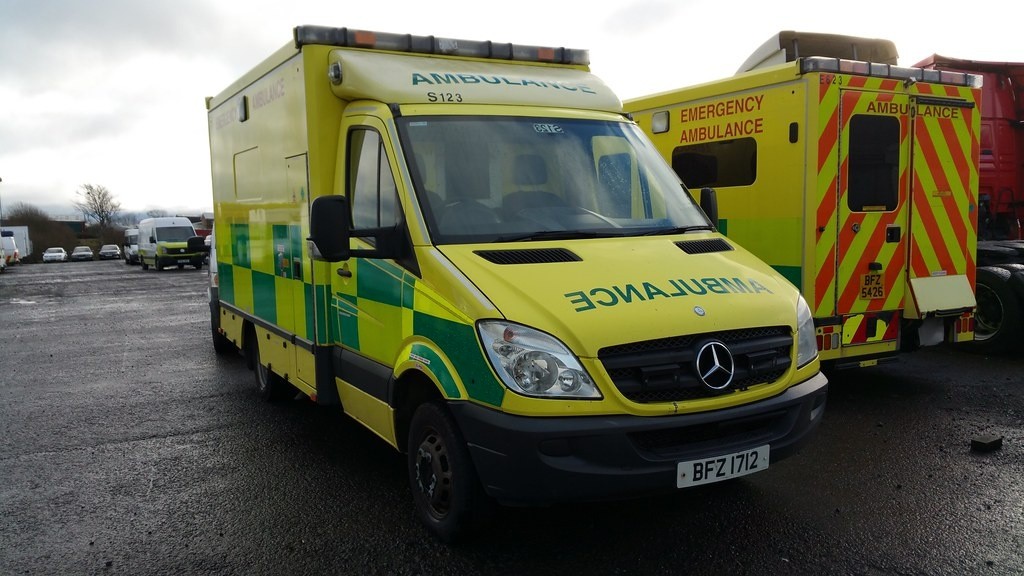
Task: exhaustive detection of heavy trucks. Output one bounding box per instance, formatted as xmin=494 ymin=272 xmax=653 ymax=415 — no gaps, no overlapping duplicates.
xmin=908 ymin=54 xmax=1024 ymax=355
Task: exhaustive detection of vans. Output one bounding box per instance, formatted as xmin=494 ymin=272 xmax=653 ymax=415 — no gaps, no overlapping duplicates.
xmin=123 ymin=229 xmax=139 ymax=264
xmin=138 ymin=217 xmax=208 ymax=272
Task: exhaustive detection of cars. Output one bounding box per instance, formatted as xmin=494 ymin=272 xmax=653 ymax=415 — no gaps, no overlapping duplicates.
xmin=42 ymin=247 xmax=68 ymax=262
xmin=1 ymin=231 xmax=20 ymax=265
xmin=187 ymin=220 xmax=223 ymax=358
xmin=0 ymin=248 xmax=6 ymax=274
xmin=98 ymin=245 xmax=121 ymax=260
xmin=70 ymin=246 xmax=95 ymax=261
xmin=0 ymin=226 xmax=33 ymax=264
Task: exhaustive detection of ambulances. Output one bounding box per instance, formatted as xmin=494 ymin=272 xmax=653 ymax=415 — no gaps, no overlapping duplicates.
xmin=619 ymin=31 xmax=984 ymax=370
xmin=205 ymin=26 xmax=830 ymax=547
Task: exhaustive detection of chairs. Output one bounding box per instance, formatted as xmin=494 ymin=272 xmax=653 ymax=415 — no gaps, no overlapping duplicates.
xmin=380 ymin=153 xmax=444 ymax=226
xmin=502 ymin=153 xmax=567 ymax=221
